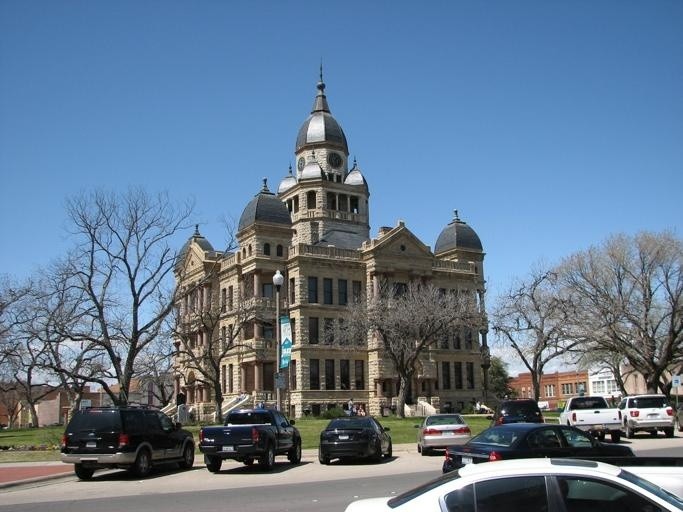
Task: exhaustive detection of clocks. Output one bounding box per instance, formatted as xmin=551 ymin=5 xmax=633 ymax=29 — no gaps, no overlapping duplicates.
xmin=329 ymin=152 xmax=342 ymax=168
xmin=297 ymin=157 xmax=305 ymax=171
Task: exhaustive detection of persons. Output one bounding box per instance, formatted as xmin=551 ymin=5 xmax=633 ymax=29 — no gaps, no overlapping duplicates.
xmin=259 ymin=401 xmax=264 ymax=409
xmin=476 ymin=400 xmax=484 ymax=414
xmin=254 ymin=402 xmax=259 ymax=409
xmin=480 ymin=400 xmax=492 ymax=413
xmin=348 ymin=397 xmax=365 ymax=417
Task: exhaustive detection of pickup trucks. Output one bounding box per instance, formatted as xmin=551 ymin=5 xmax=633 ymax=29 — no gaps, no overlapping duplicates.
xmin=558 ymin=395 xmax=622 ymax=443
xmin=199 ymin=408 xmax=301 ymax=472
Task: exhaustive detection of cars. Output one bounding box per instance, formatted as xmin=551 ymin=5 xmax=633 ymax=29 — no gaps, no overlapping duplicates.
xmin=344 ymin=457 xmax=683 ymax=512
xmin=415 ymin=413 xmax=471 ymax=456
xmin=319 ymin=416 xmax=392 ymax=464
xmin=676 ymin=402 xmax=683 ymax=432
xmin=60 ymin=403 xmax=195 ymax=480
xmin=487 ymin=398 xmax=544 ymax=427
xmin=615 ymin=394 xmax=676 ymax=438
xmin=443 ymin=422 xmax=636 ymax=474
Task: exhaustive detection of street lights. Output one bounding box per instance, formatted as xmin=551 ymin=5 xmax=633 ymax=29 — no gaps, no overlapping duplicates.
xmin=272 ymin=269 xmax=284 ymax=411
